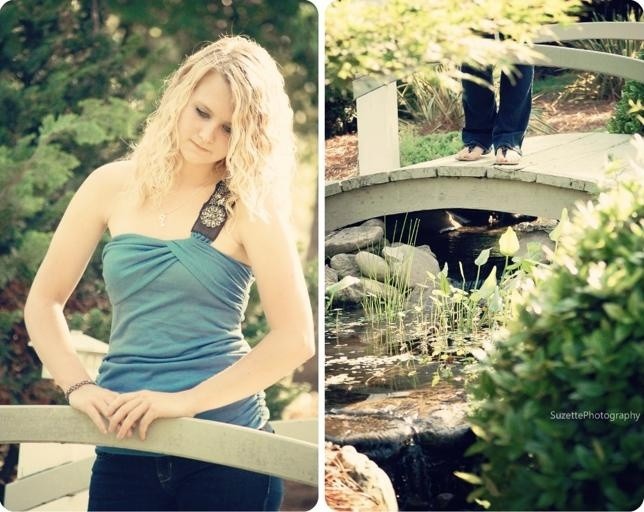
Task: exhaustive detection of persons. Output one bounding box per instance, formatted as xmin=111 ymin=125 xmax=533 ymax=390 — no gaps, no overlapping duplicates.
xmin=456 ymin=7 xmax=535 ymax=168
xmin=21 ymin=33 xmax=318 ymax=511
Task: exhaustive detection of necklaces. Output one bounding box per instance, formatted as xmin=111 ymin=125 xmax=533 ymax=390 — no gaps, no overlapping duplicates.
xmin=150 ymin=163 xmax=224 ymax=225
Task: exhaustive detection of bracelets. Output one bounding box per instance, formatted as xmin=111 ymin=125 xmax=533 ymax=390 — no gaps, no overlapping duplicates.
xmin=64 ymin=379 xmax=97 ymax=405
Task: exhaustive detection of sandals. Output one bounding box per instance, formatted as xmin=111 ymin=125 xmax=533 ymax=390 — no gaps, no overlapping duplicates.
xmin=456 ymin=145 xmax=490 ymax=160
xmin=494 ymin=150 xmax=520 ymax=166
